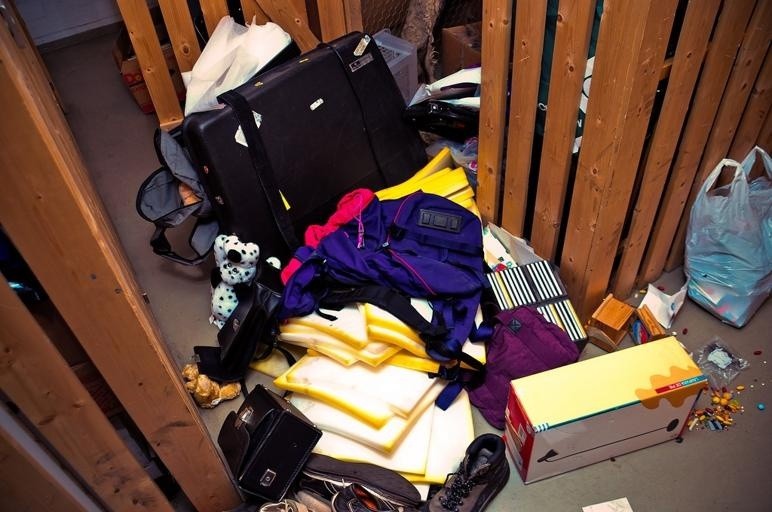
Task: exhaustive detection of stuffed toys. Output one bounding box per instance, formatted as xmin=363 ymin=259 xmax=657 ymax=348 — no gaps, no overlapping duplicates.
xmin=209 ymin=233 xmax=261 ymax=329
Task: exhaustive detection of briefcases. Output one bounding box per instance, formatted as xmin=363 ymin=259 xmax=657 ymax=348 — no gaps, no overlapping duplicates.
xmin=171 ymin=25 xmax=433 ymax=278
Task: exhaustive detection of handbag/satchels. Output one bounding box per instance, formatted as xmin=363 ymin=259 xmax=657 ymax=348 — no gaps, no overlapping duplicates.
xmin=463 ymin=302 xmax=581 ymax=434
xmin=215 ymin=383 xmax=324 ymax=507
xmin=187 ymin=275 xmax=297 ymax=400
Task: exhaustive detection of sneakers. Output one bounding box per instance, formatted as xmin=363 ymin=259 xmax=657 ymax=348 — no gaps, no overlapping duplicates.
xmin=418 ymin=434 xmax=511 ymax=512
xmin=330 ymin=484 xmax=401 ymax=512
xmin=297 ymin=453 xmax=420 ymax=512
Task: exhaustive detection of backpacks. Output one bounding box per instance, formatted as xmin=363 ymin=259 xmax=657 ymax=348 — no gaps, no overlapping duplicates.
xmin=279 ymin=187 xmax=496 ymax=411
xmin=135 ymin=126 xmax=220 ymax=267
